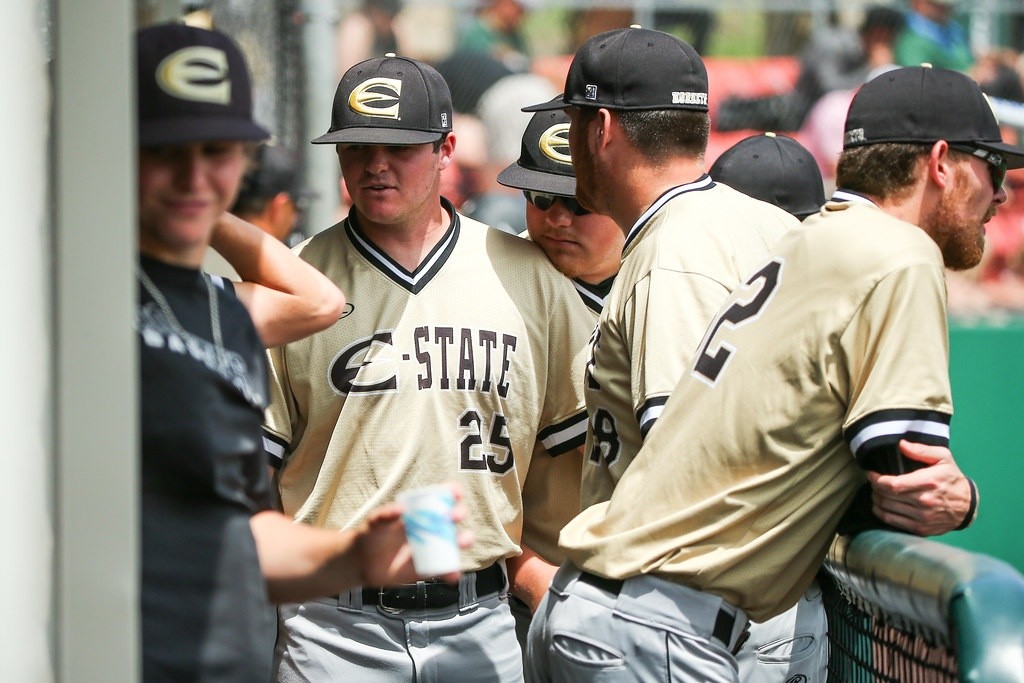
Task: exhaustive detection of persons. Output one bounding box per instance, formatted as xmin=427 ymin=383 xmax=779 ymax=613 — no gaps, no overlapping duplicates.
xmin=521 ymin=63 xmax=1024 ymax=683
xmin=136 ymin=21 xmax=474 ymax=683
xmin=792 ymin=0 xmax=1024 ymax=109
xmin=224 ymin=0 xmax=832 ymax=683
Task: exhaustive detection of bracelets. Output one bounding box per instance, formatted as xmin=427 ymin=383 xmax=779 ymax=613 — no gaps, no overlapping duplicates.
xmin=954 ymin=477 xmax=976 ymax=530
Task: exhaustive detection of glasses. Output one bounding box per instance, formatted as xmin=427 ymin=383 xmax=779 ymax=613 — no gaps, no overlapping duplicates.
xmin=523 ymin=190 xmax=592 ymax=216
xmin=949 ymin=143 xmax=1008 ymax=194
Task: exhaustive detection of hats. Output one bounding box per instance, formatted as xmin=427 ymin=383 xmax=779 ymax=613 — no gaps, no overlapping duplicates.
xmin=310 ymin=51 xmax=453 ymax=145
xmin=495 ymin=92 xmax=577 ymax=197
xmin=137 ymin=20 xmax=272 ymax=150
xmin=521 ymin=25 xmax=709 ymax=112
xmin=708 ymin=132 xmax=828 ymax=215
xmin=843 ymin=62 xmax=1024 ymax=170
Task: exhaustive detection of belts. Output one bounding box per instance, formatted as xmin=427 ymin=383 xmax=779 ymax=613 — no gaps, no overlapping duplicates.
xmin=362 ymin=562 xmax=506 ymax=614
xmin=577 ymin=570 xmax=751 ymax=657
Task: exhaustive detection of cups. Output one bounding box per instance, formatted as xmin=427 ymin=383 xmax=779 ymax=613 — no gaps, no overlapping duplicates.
xmin=394 ymin=483 xmax=461 ymax=577
